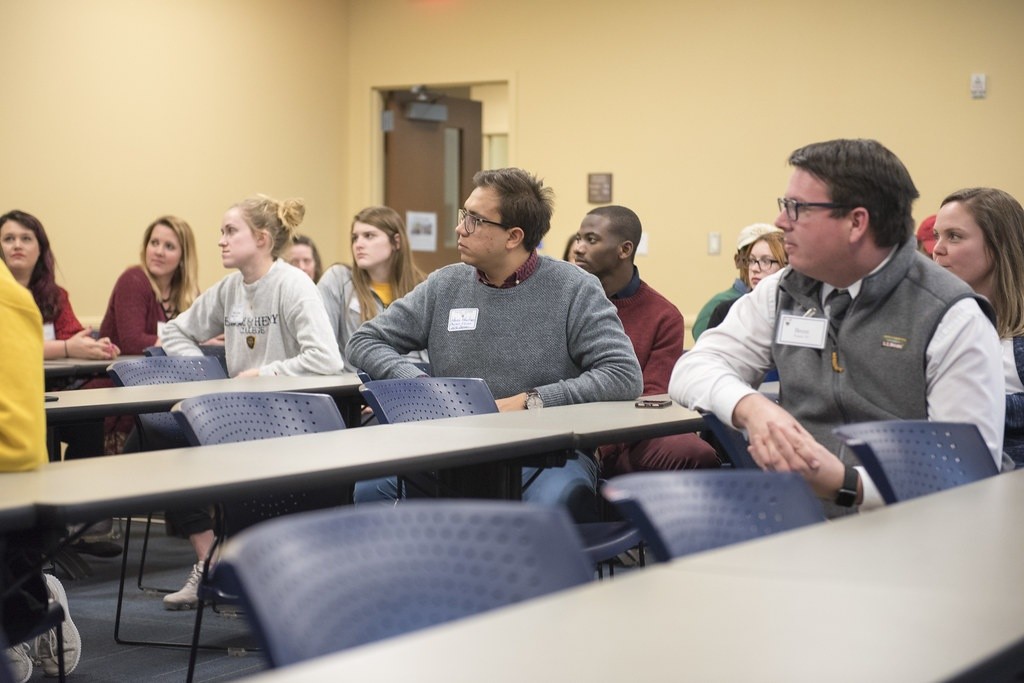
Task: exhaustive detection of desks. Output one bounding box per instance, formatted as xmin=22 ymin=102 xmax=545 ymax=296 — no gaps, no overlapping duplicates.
xmin=42 ymin=373 xmax=370 ymax=413
xmin=35 ymin=348 xmax=157 ymax=372
xmin=0 ymin=376 xmax=802 ymax=520
xmin=221 ymin=466 xmax=1024 ymax=683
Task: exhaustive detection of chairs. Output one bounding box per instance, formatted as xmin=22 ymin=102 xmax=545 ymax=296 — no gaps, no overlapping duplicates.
xmin=0 ymin=320 xmax=1024 ymax=683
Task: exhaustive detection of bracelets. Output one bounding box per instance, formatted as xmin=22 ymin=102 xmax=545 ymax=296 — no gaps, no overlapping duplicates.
xmin=64 ymin=340 xmax=68 ymax=357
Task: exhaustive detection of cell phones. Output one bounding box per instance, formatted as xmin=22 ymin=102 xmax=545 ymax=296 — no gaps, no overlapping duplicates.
xmin=636 ymin=400 xmax=673 ymax=407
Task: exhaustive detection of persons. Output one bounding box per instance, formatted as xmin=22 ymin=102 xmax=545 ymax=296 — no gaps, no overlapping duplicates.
xmin=278 ymin=235 xmax=322 ymax=284
xmin=160 ymin=193 xmax=344 ymax=609
xmin=706 ymin=231 xmax=789 ymax=382
xmin=0 ymin=256 xmax=81 ymax=683
xmin=98 ymin=215 xmax=225 ymax=356
xmin=344 ymin=165 xmax=643 ymax=504
xmin=917 ymin=214 xmax=936 ymax=261
xmin=572 ymin=205 xmax=721 ymax=477
xmin=0 ymin=209 xmax=124 ymax=558
xmin=692 ymin=223 xmax=783 ymax=342
xmin=315 ymin=206 xmax=430 ymax=373
xmin=667 ymin=138 xmax=1007 ymax=519
xmin=933 ymin=187 xmax=1024 ymax=472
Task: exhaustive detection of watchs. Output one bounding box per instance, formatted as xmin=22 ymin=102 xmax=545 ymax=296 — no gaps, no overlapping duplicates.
xmin=524 ymin=389 xmax=544 ymax=410
xmin=834 ymin=463 xmax=859 ymax=508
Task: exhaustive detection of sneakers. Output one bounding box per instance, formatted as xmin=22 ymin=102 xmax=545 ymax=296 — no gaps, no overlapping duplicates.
xmin=0 ymin=641 xmax=33 ymax=683
xmin=35 ymin=574 xmax=81 ymax=675
xmin=163 ymin=560 xmax=216 ymax=609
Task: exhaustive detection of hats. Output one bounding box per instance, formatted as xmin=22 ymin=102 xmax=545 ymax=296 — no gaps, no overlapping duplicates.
xmin=736 ymin=223 xmax=784 ymax=250
xmin=917 ymin=215 xmax=939 ymax=254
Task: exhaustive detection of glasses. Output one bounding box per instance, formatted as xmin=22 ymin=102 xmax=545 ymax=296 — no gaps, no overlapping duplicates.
xmin=777 ymin=198 xmax=858 ymax=221
xmin=457 ymin=207 xmax=514 ymax=234
xmin=743 ymin=257 xmax=788 ymax=271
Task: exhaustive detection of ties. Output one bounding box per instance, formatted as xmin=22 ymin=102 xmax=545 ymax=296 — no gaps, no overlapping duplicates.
xmin=823 ymin=290 xmax=848 ymax=347
